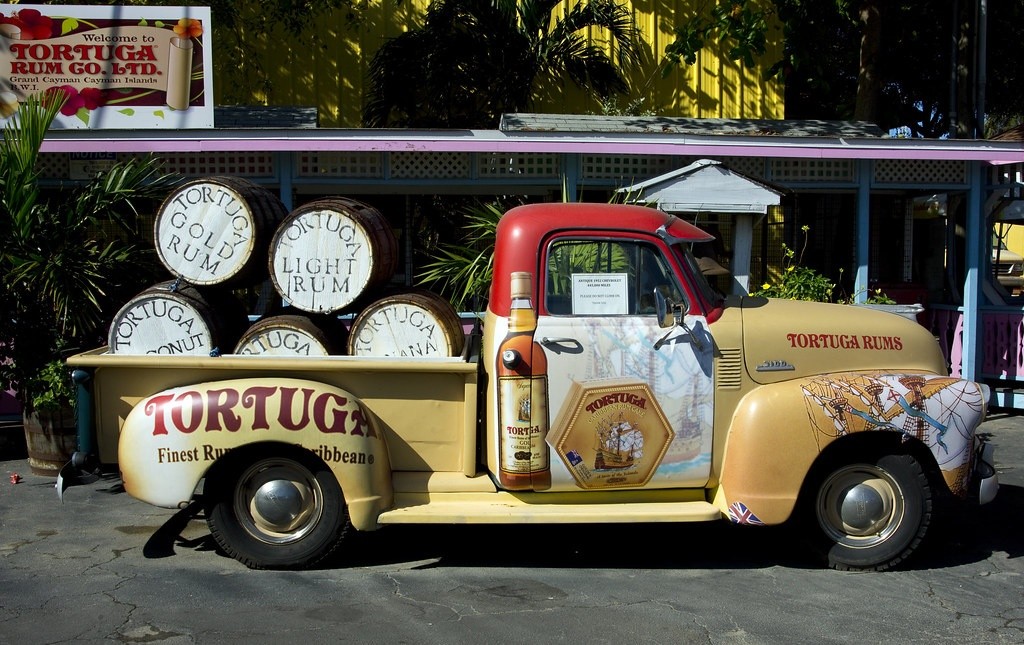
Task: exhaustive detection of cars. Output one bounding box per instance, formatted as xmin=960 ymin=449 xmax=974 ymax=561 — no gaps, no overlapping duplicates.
xmin=930 ymin=184 xmax=1024 ymax=300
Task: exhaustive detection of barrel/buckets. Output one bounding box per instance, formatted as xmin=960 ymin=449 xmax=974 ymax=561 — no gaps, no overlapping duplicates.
xmin=108 ymin=173 xmax=465 ymax=357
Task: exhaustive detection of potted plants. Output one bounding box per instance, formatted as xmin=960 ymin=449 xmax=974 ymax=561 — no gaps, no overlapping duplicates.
xmin=0 ymin=87 xmax=187 ymax=477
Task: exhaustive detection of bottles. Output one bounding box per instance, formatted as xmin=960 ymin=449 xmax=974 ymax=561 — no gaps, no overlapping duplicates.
xmin=495 ymin=272 xmax=552 ymax=490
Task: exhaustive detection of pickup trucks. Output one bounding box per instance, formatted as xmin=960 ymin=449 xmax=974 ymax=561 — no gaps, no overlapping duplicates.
xmin=65 ymin=202 xmax=997 ymax=572
